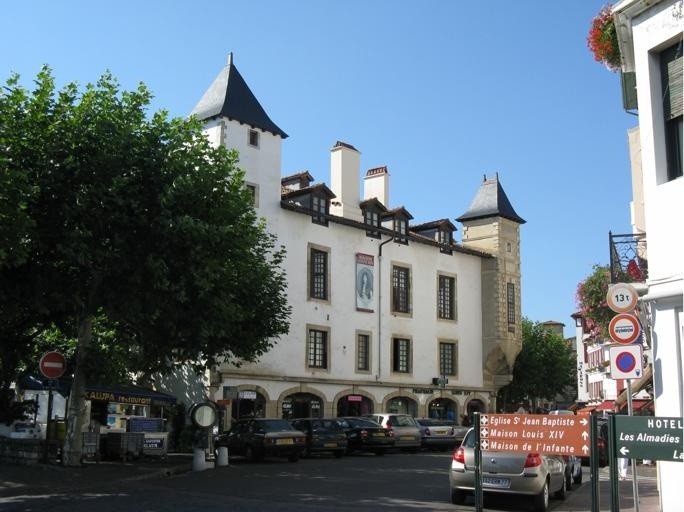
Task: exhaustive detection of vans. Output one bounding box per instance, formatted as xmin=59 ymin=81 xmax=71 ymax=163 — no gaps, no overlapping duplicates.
xmin=0 ymin=419 xmax=42 ymax=439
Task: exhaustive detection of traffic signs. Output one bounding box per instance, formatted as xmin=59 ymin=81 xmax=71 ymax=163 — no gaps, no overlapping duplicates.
xmin=480 ymin=415 xmax=592 ymax=457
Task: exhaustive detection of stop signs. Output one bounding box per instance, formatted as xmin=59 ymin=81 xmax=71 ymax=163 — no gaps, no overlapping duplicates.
xmin=40 ymin=351 xmax=66 ymax=380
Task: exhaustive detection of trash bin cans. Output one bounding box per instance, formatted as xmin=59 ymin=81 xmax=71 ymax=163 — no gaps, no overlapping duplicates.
xmin=192 ymin=447 xmax=206 ymax=473
xmin=216 ymin=446 xmax=228 ymax=466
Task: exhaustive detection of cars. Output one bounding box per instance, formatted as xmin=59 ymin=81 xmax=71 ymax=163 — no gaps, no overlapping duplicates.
xmin=414 ymin=418 xmax=457 ymax=452
xmin=449 ymin=428 xmax=568 ymax=509
xmin=445 ymin=420 xmax=469 ymax=443
xmin=289 ymin=418 xmax=349 ymax=458
xmin=365 ymin=414 xmax=421 ymax=452
xmin=244 ymin=417 xmax=308 ymax=462
xmin=565 ymin=456 xmax=584 ymax=489
xmin=343 ymin=417 xmax=395 ymax=456
xmin=584 ymin=419 xmax=608 ymax=466
